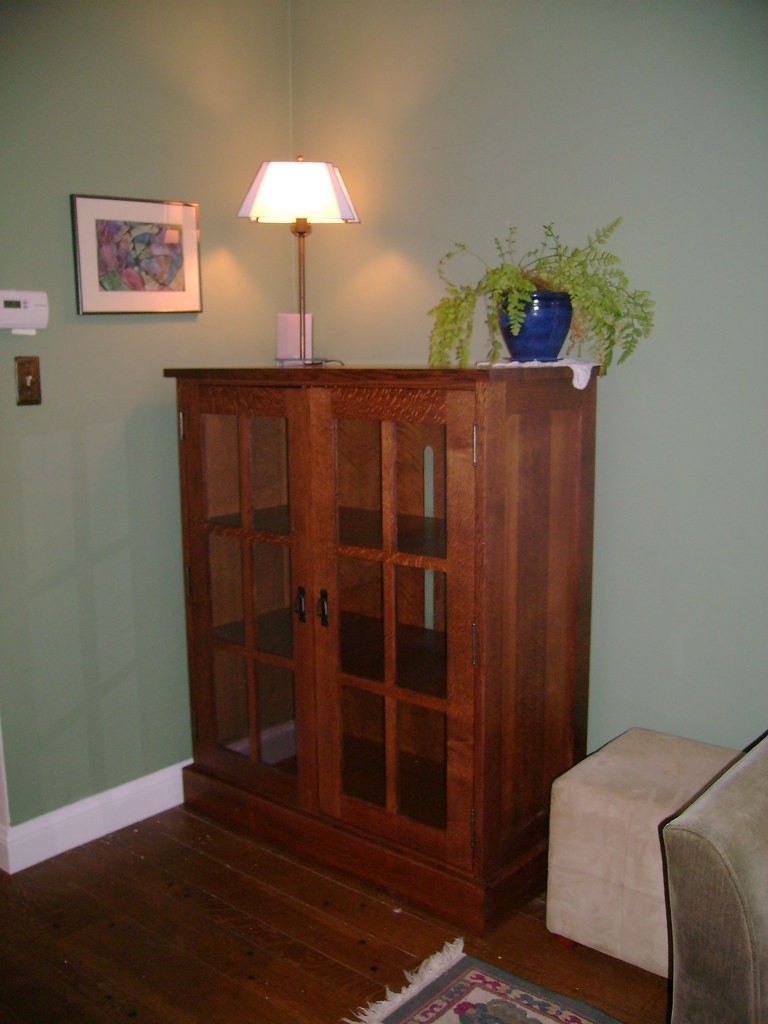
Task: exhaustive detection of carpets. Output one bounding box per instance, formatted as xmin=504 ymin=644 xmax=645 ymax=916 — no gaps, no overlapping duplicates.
xmin=340 ymin=938 xmax=624 ymax=1024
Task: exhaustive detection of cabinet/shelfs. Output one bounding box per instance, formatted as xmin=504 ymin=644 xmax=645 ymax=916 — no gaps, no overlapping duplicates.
xmin=161 ymin=364 xmax=601 ymax=941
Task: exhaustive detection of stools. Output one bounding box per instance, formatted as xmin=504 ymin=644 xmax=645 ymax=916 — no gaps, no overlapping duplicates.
xmin=545 ymin=726 xmax=747 ymax=979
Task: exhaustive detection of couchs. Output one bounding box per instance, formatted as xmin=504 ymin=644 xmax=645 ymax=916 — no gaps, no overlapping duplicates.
xmin=662 ymin=735 xmax=768 ymax=1024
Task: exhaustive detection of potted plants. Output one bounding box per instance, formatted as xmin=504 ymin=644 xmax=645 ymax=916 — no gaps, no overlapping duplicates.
xmin=426 ymin=215 xmax=654 ymax=377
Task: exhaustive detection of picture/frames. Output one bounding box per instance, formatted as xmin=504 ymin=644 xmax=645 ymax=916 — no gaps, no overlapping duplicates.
xmin=70 ymin=193 xmax=204 ymax=315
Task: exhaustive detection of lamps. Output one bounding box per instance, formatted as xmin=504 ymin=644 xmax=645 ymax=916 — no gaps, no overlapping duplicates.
xmin=237 ymin=156 xmax=362 ymax=367
